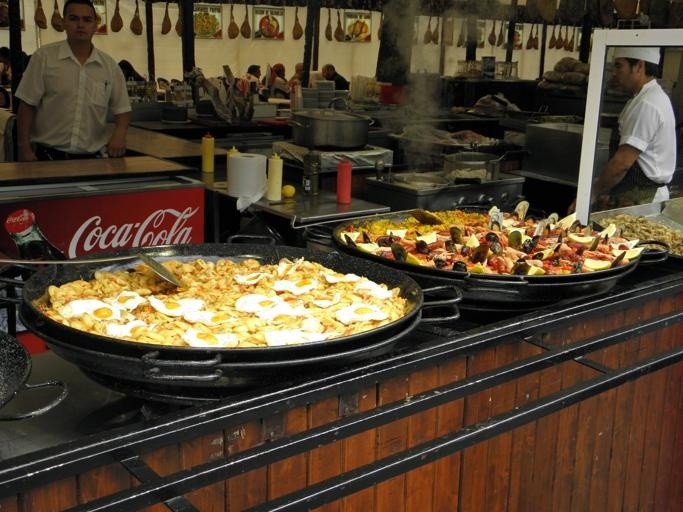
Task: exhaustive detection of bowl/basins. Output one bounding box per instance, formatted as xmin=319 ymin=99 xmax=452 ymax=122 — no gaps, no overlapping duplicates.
xmin=276 ymin=108 xmax=291 ymax=117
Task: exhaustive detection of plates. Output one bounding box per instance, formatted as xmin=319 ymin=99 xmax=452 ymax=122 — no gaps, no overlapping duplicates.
xmin=272 ymin=116 xmax=291 ymax=121
xmin=300 ymin=80 xmax=349 ymax=110
xmin=162 ymin=119 xmax=192 ymax=125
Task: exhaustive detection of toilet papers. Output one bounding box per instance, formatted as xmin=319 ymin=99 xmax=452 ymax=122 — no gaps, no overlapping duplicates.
xmin=227 ymin=152 xmax=267 ymax=212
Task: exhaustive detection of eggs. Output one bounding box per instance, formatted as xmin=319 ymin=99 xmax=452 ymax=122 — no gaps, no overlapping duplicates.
xmin=58 ymin=255 xmax=393 ymax=347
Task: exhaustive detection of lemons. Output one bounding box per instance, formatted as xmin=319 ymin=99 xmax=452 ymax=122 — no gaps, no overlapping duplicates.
xmin=282 ymin=185 xmax=295 ymax=197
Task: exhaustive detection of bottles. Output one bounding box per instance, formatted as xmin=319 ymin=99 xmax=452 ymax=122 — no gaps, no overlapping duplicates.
xmin=267 ymin=153 xmax=283 ymax=202
xmin=5 ymin=208 xmax=70 ymax=282
xmin=201 ymin=131 xmax=215 ymax=173
xmin=303 ymin=151 xmax=320 ymax=196
xmin=336 ymin=156 xmax=353 ymax=205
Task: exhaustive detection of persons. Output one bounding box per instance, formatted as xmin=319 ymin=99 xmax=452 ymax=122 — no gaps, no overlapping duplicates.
xmin=13 ymin=0 xmax=130 ymax=162
xmin=0 ymin=47 xmax=147 ymax=99
xmin=241 ymin=63 xmax=349 ymax=99
xmin=568 ymin=58 xmax=677 ymax=216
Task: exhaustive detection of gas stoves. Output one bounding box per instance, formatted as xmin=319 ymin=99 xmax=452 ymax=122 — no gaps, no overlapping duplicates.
xmin=364 ymin=169 xmax=527 ymax=211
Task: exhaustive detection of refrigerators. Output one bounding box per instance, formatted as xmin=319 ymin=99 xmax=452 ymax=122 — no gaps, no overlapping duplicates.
xmin=0 ymin=174 xmax=206 ymax=354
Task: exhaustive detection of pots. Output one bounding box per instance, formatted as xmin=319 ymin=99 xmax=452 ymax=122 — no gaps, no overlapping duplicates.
xmin=1 ymin=329 xmax=71 ymax=422
xmin=303 ymin=208 xmax=671 ymax=303
xmin=0 ymin=234 xmax=464 ymax=389
xmin=440 ymin=151 xmax=505 ymax=181
xmin=287 ymin=108 xmax=375 ymax=151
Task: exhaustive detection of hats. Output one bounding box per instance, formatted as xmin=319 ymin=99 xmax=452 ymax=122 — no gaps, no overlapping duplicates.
xmin=614 ymin=47 xmax=661 ymax=65
